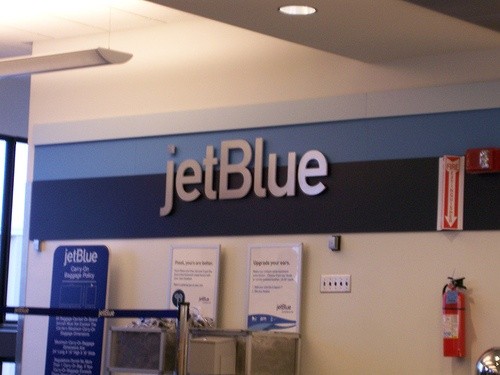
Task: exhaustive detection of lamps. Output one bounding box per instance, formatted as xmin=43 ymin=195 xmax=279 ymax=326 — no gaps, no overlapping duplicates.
xmin=1 ymin=6 xmax=131 ymax=79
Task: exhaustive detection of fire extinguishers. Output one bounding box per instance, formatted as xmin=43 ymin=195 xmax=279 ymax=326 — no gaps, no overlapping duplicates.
xmin=442 ymin=275 xmax=468 ymax=358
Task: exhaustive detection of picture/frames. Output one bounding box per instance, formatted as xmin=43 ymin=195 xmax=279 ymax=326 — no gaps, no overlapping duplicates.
xmin=168 ymin=244 xmax=222 ymax=330
xmin=244 ymin=243 xmax=302 ymax=336
